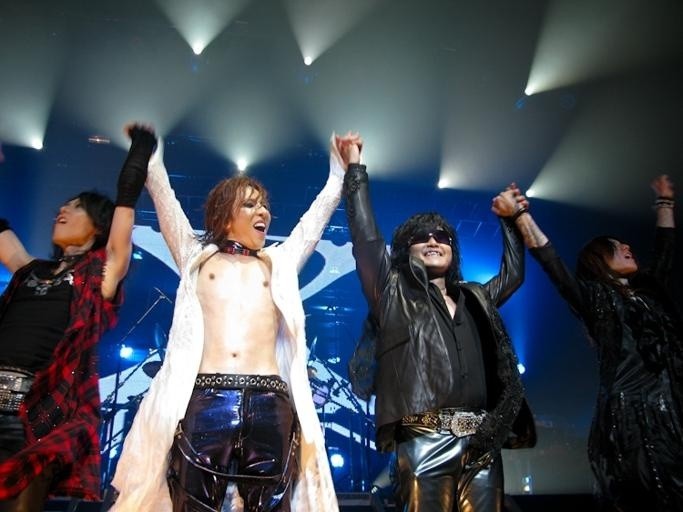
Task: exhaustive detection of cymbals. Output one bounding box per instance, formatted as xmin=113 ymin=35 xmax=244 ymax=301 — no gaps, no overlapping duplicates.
xmin=143 ymin=359 xmax=163 ymax=378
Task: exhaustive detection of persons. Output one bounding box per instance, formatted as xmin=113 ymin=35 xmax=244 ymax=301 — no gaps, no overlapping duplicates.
xmin=107 ymin=120 xmax=362 ymax=512
xmin=490 ymin=174 xmax=683 ymax=512
xmin=0 ymin=120 xmax=157 ymax=512
xmin=342 ymin=130 xmax=537 ymax=512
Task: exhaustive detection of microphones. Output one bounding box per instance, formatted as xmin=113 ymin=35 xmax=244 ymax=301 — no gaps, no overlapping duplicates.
xmin=154 ymin=286 xmax=172 ymax=303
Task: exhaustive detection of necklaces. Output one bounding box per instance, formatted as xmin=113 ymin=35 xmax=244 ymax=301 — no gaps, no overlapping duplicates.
xmin=220 ymin=240 xmax=257 ymax=257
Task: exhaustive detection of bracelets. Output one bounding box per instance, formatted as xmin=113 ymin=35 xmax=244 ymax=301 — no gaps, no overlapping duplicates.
xmin=510 ymin=206 xmax=528 ymax=224
xmin=654 ymin=196 xmax=675 ymax=209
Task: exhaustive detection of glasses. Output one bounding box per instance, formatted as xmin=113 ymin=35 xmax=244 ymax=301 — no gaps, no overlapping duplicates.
xmin=408 ymin=229 xmax=451 ymax=245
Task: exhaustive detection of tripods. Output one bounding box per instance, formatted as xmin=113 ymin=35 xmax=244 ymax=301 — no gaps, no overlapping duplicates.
xmin=97 ymin=299 xmax=165 ymax=512
xmin=306 ymin=352 xmax=377 ymax=493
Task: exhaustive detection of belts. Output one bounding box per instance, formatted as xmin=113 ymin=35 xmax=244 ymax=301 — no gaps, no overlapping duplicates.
xmin=193 ymin=372 xmax=290 ymax=398
xmin=398 ymin=407 xmax=486 ymax=438
xmin=0 ymin=375 xmax=35 ymax=415
